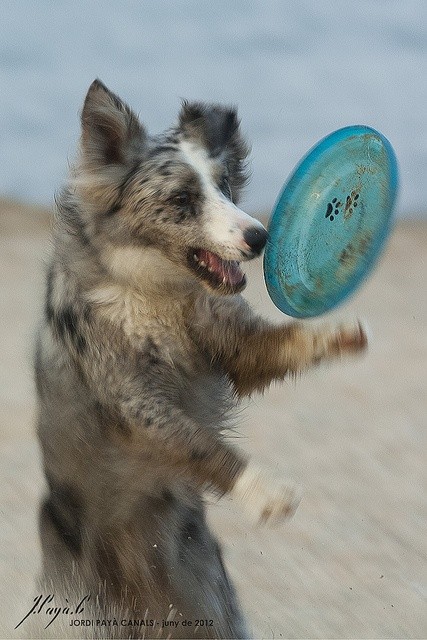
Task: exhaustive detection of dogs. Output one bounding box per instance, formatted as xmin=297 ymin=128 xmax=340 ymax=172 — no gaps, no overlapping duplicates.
xmin=34 ymin=79 xmax=368 ymax=638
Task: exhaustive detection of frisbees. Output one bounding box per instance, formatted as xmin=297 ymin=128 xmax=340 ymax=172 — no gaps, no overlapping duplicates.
xmin=262 ymin=125 xmax=399 ymax=320
